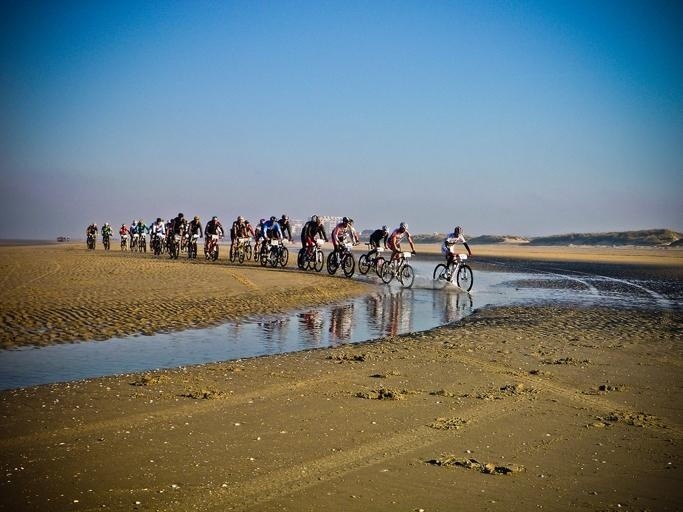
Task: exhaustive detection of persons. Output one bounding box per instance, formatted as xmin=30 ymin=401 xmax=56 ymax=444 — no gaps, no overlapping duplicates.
xmin=87 ymin=223 xmax=98 ymax=249
xmin=440 ymin=224 xmax=472 ymax=279
xmin=387 ymin=222 xmax=415 ymax=266
xmin=230 ymin=214 xmax=292 ymax=257
xmin=205 ymin=216 xmax=224 ymax=253
xmin=365 ymin=225 xmax=389 ymax=261
xmin=301 ymin=215 xmax=359 ymax=266
xmin=101 ymin=222 xmax=112 ymax=248
xmin=119 ymin=219 xmax=149 ymax=248
xmin=149 ymin=213 xmax=203 ymax=254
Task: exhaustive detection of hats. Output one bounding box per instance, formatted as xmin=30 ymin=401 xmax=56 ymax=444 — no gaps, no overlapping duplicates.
xmin=343 ymin=217 xmax=353 ymax=222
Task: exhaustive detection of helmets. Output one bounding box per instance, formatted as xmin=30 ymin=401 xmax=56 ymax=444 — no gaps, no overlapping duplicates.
xmin=238 ymin=216 xmax=244 ymax=219
xmin=455 ymin=226 xmax=463 ymax=234
xmin=260 ymin=214 xmax=290 ymax=224
xmin=214 ymin=217 xmax=218 ymax=225
xmin=400 ymin=222 xmax=409 ymax=229
xmin=383 ymin=225 xmax=389 ymax=231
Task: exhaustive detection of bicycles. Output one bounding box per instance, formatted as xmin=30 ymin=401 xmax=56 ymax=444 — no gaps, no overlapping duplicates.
xmin=87 ymin=232 xmax=225 ymax=261
xmin=379 ymin=280 xmax=416 ymax=313
xmin=380 ymin=247 xmax=417 ymax=289
xmin=297 ymin=240 xmax=361 ymax=278
xmin=432 ymin=253 xmax=476 ymax=293
xmin=429 ymin=294 xmax=477 ymax=314
xmin=358 ymin=242 xmax=387 ymax=278
xmin=229 ymin=233 xmax=294 ymax=268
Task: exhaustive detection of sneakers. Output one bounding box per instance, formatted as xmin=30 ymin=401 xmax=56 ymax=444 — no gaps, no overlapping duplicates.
xmin=446 ymin=268 xmax=451 ymax=275
